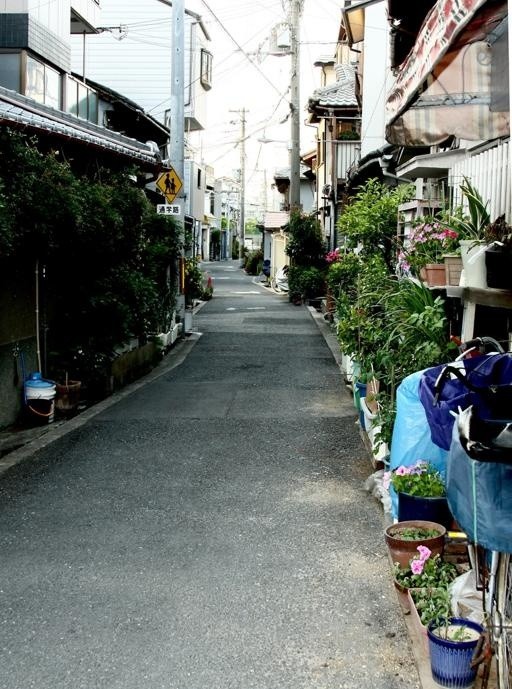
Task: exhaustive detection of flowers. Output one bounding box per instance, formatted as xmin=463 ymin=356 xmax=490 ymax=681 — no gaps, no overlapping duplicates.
xmin=396 ymin=224 xmax=459 ymax=272
xmin=391 ymin=459 xmax=446 ymax=496
xmin=394 ymin=545 xmax=457 ymax=587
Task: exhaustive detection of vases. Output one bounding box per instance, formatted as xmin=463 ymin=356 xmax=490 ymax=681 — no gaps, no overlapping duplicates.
xmin=443 ymin=252 xmax=463 ymax=286
xmin=485 ymin=249 xmax=512 ymax=289
xmin=394 ymin=574 xmax=410 ymax=610
xmin=398 ymin=492 xmax=452 ymax=533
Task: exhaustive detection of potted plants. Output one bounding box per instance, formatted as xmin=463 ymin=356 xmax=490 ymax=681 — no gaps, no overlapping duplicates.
xmin=434 ymin=174 xmax=487 ymax=287
xmin=408 ymin=576 xmax=486 ymax=688
xmin=326 ymin=234 xmax=450 ymax=432
xmin=384 ymin=520 xmax=446 ymax=569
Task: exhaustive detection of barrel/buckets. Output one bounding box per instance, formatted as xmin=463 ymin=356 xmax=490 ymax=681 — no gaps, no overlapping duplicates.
xmin=24 ymin=380 xmax=57 ymax=423
xmin=28 ymin=398 xmax=54 ymax=425
